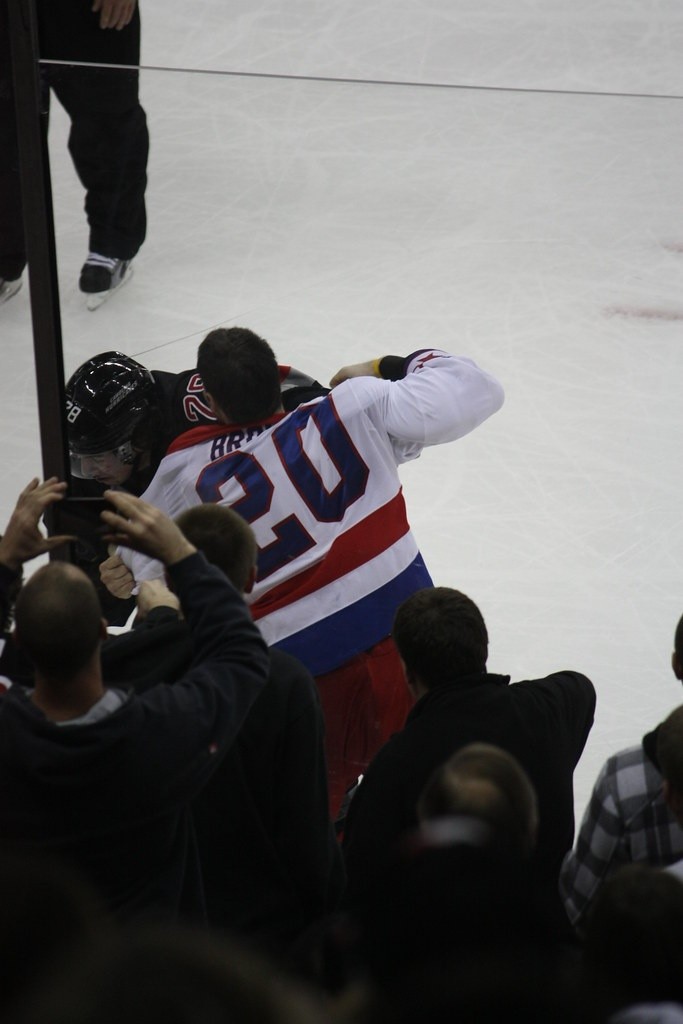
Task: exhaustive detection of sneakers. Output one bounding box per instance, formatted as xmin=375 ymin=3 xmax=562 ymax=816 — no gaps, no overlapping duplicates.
xmin=78 ymin=251 xmax=137 ymax=311
xmin=0 ymin=273 xmax=23 ymax=305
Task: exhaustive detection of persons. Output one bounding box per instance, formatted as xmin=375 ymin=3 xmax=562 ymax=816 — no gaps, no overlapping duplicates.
xmin=0 ymin=326 xmax=683 ymax=1024
xmin=0 ymin=0 xmax=149 ymax=310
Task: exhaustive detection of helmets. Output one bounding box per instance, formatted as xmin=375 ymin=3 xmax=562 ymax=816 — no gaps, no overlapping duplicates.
xmin=64 ymin=350 xmax=152 ymax=456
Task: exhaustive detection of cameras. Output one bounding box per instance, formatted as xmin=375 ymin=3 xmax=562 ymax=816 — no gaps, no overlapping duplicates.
xmin=44 ymin=495 xmax=119 ymax=540
